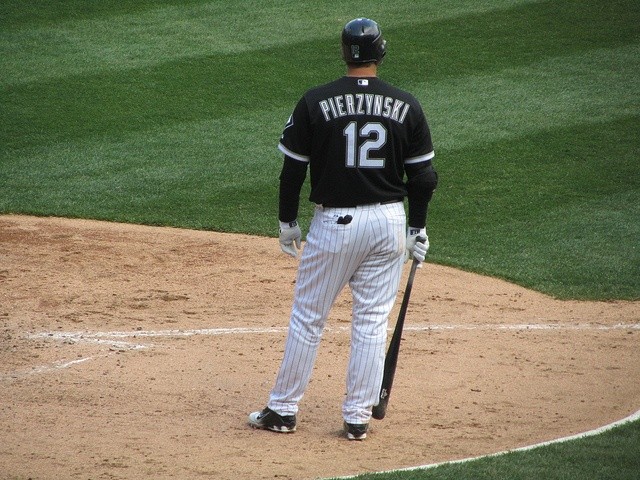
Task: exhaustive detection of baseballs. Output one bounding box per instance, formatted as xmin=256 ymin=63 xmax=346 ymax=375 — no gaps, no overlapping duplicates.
xmin=373 ymin=236 xmax=427 ymax=420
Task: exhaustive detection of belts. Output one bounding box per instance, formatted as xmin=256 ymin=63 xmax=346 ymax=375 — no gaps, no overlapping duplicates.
xmin=324 ymin=197 xmax=403 ymax=209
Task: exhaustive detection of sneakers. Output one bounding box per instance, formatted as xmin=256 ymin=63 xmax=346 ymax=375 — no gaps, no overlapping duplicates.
xmin=248 ymin=407 xmax=296 ymax=433
xmin=344 ymin=420 xmax=368 ymax=439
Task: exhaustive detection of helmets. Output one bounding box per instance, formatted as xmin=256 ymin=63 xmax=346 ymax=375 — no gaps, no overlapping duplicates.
xmin=340 ymin=18 xmax=387 ymax=64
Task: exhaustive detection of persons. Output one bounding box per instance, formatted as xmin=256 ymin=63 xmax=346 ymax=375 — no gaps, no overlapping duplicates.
xmin=249 ymin=19 xmax=438 ymax=440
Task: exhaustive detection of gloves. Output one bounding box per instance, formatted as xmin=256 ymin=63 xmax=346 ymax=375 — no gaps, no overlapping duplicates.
xmin=404 ymin=226 xmax=429 ymax=268
xmin=279 ymin=219 xmax=301 ymax=257
